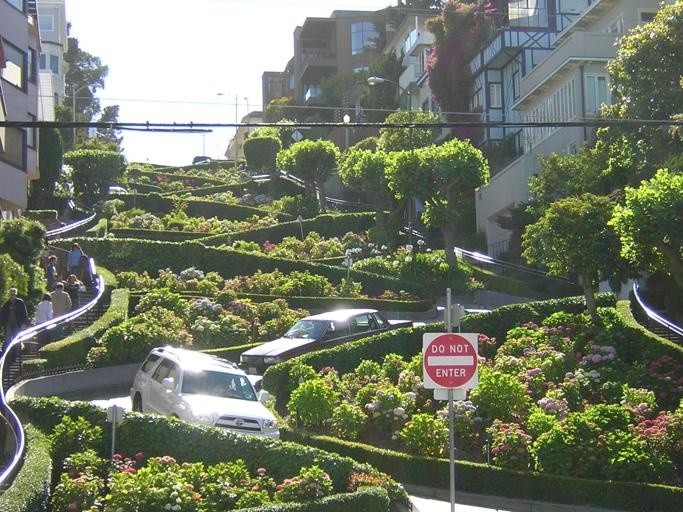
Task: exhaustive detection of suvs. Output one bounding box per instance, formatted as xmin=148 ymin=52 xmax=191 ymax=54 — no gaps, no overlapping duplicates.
xmin=129 ymin=344 xmax=280 ymax=439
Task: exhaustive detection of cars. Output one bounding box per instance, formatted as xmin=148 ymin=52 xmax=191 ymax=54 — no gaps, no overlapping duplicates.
xmin=239 ymin=307 xmax=414 ymax=376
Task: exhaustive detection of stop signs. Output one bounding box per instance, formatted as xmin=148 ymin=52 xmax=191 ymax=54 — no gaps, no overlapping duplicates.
xmin=423 ymin=333 xmax=478 ymax=388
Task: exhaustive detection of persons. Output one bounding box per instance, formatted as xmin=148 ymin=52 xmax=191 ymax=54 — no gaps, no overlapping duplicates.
xmin=0 ymin=242 xmax=84 ymax=350
xmin=63 ymin=179 xmax=70 ymax=192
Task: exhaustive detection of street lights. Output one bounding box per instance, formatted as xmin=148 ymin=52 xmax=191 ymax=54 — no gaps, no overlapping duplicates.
xmin=367 ymin=75 xmax=414 ymax=246
xmin=217 ymin=92 xmax=239 ymax=170
xmin=343 ymin=114 xmax=352 ymax=151
